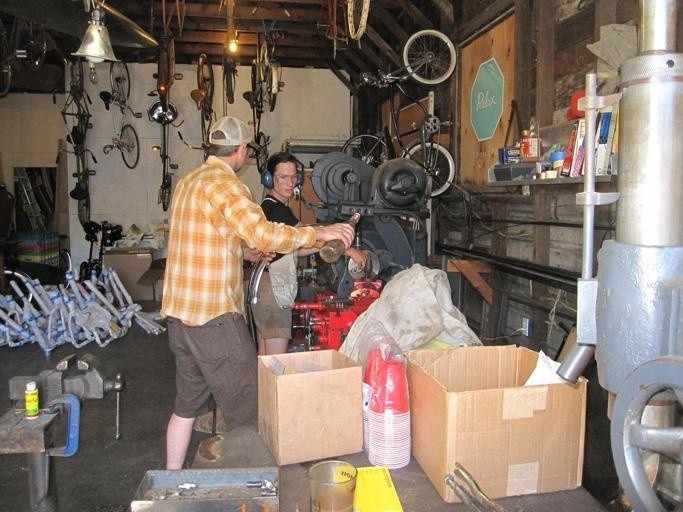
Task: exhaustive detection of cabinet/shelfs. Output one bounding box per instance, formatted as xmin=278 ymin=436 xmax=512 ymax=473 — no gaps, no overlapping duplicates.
xmin=430 ymin=185 xmax=619 ymax=362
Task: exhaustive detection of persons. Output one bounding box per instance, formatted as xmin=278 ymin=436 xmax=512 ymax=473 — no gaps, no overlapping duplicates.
xmin=247 ymin=150 xmax=369 ymax=357
xmin=158 ymin=115 xmax=357 ymax=470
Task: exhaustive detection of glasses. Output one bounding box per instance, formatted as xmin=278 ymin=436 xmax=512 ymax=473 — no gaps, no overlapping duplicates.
xmin=276 ymin=174 xmax=301 ymax=184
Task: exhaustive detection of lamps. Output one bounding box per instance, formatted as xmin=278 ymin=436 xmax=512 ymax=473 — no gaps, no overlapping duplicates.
xmin=69 ymin=0 xmax=159 ymax=65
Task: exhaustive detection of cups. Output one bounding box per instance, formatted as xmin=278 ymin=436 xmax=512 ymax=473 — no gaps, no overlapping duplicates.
xmin=362 ymin=347 xmax=411 ymax=470
xmin=307 ymin=459 xmax=358 ymax=512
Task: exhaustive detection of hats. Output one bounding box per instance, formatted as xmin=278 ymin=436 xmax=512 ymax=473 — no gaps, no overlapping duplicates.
xmin=208 ymin=116 xmax=251 ymax=146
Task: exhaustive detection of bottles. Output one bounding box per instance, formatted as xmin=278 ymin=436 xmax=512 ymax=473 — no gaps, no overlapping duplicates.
xmin=319 ymin=212 xmax=362 ymax=264
xmin=23 ymin=381 xmax=40 ymax=420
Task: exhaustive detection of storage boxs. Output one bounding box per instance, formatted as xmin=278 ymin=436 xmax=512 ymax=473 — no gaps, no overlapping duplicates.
xmin=403 ymin=343 xmax=590 ymax=504
xmin=257 ymin=349 xmax=364 ymax=468
xmin=103 ymin=247 xmax=167 ymax=302
